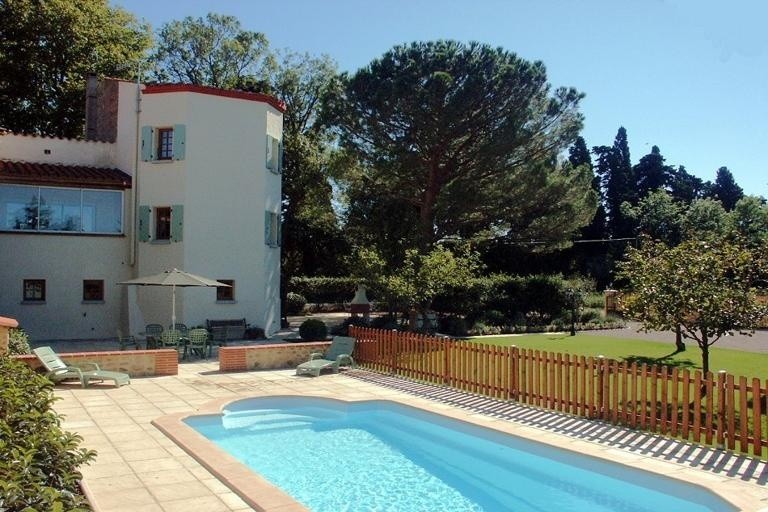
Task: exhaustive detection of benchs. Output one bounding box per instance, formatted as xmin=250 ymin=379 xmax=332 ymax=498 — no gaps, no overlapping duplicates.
xmin=206 ymin=318 xmax=256 ymax=354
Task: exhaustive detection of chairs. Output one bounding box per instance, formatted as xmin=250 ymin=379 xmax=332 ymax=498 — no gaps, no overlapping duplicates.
xmin=115 ymin=323 xmax=228 ymax=362
xmin=31 ymin=346 xmax=131 ymax=389
xmin=297 ymin=335 xmax=358 ymax=378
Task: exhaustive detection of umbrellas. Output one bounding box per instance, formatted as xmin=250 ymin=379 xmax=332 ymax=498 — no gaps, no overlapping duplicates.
xmin=116 ymin=267 xmax=233 ymax=330
